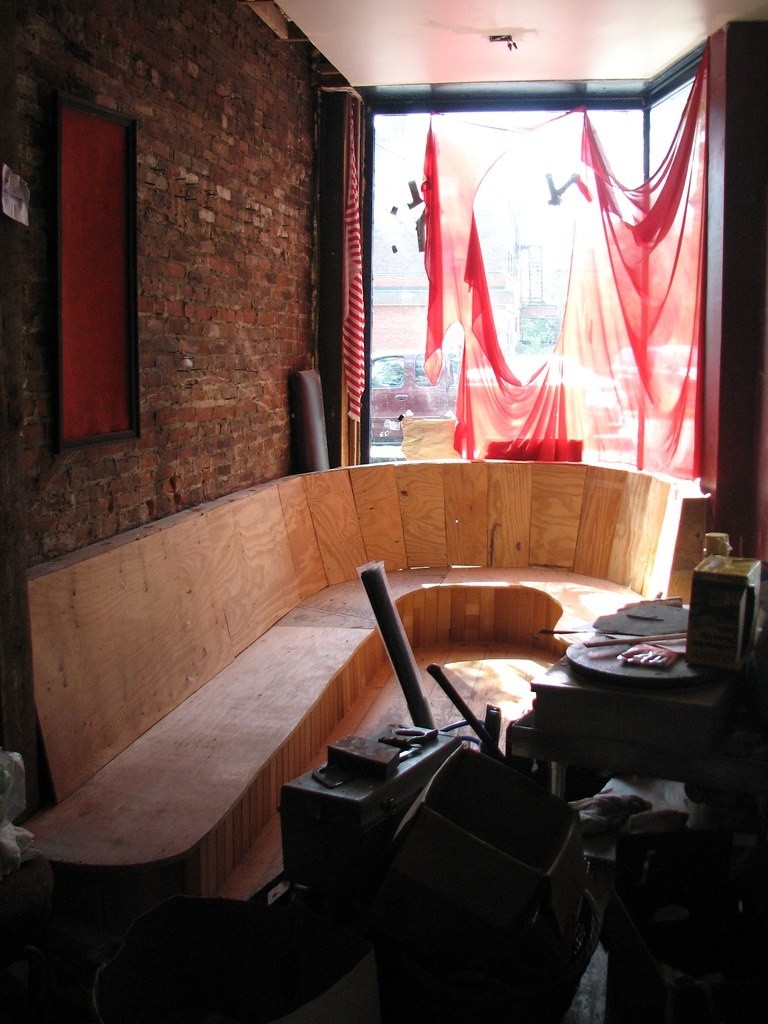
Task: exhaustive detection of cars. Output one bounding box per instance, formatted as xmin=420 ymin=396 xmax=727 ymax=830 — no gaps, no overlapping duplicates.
xmin=370 ymin=349 xmax=463 ymax=446
xmin=515 ymin=305 xmax=559 ymax=355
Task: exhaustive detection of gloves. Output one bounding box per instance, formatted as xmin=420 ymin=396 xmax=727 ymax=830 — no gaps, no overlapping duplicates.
xmin=618 ymin=643 xmax=679 ymax=670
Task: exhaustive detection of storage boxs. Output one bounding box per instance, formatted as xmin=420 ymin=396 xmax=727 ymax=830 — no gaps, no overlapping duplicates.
xmin=280 ymin=723 xmax=459 ymax=895
xmin=369 ymin=746 xmax=588 ymax=968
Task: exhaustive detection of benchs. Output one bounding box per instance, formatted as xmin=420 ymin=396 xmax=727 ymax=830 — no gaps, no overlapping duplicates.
xmin=8 ymin=454 xmax=653 ymax=899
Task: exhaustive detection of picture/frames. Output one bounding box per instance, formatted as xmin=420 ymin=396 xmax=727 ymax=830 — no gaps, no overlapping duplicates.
xmin=48 ymin=86 xmax=140 ymax=451
